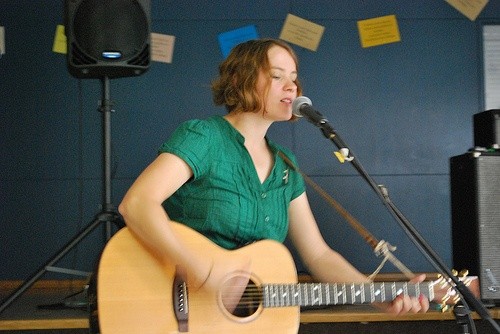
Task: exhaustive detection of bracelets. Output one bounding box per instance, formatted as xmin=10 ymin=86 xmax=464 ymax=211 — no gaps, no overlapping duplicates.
xmin=197 ymin=264 xmax=213 ymax=294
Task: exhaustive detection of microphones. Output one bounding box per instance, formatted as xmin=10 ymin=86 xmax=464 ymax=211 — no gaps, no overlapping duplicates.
xmin=292 ymin=96 xmax=327 ymax=127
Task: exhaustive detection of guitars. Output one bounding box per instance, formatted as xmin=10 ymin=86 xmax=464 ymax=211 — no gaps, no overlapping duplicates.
xmin=88 ymin=219 xmax=479 ymax=334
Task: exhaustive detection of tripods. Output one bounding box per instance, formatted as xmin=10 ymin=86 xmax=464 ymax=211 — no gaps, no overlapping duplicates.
xmin=1 ymin=78 xmax=131 ymax=314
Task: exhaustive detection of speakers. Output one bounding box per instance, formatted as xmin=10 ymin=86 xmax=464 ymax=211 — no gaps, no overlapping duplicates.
xmin=450 ymin=152 xmax=500 ymax=309
xmin=65 ymin=0 xmax=151 ymax=79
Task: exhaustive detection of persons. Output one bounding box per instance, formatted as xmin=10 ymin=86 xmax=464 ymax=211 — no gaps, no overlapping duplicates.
xmin=118 ymin=38 xmax=430 ymax=322
